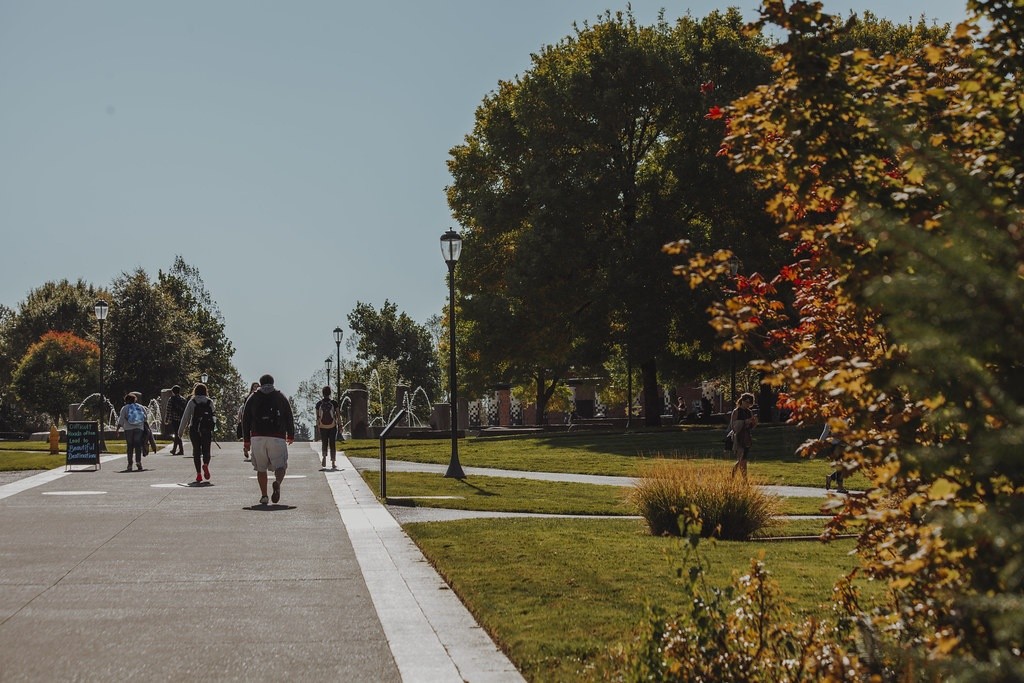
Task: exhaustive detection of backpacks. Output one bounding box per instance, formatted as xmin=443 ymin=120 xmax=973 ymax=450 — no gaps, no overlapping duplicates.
xmin=125 ymin=403 xmax=145 ymax=425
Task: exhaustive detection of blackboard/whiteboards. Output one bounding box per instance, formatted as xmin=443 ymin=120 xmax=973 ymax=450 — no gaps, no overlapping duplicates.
xmin=66 ymin=421 xmax=100 ymax=465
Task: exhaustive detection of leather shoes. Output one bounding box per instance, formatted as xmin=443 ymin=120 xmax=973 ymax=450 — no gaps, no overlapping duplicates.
xmin=170 ymin=450 xmax=184 ymax=455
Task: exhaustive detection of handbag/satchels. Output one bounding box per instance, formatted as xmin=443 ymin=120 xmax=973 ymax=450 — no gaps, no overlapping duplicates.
xmin=726 ymin=430 xmax=736 ymax=451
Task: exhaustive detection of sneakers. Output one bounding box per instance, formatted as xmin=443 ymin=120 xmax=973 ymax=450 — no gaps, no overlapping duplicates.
xmin=261 ymin=481 xmax=280 ymax=503
xmin=195 ymin=464 xmax=210 ymax=482
xmin=127 ymin=461 xmax=142 ymax=472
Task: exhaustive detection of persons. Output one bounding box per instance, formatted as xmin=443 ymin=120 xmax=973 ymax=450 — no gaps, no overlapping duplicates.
xmin=116 ymin=394 xmax=148 ymax=472
xmin=177 ymin=382 xmax=215 ymax=480
xmin=676 ymin=397 xmax=686 ymax=425
xmin=243 ymin=375 xmax=295 ymax=504
xmin=820 ymin=422 xmax=849 ymax=493
xmin=238 ymin=382 xmax=259 ymax=457
xmin=732 ymin=393 xmax=758 ymax=485
xmin=315 ymin=386 xmax=342 ymax=469
xmin=165 ymin=385 xmax=187 ymax=455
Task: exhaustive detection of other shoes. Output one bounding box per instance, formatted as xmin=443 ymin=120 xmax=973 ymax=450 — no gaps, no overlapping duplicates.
xmin=322 ymin=457 xmax=326 ymax=467
xmin=332 ymin=465 xmax=337 ymax=468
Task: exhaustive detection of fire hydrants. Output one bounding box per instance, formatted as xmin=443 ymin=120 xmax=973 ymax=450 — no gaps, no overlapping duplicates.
xmin=49 ymin=424 xmax=60 ymax=454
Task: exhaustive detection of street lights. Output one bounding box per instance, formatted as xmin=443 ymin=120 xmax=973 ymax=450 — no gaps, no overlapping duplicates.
xmin=94 ymin=299 xmax=108 ymax=451
xmin=441 ymin=226 xmax=465 ymax=476
xmin=325 ymin=359 xmax=332 ymax=386
xmin=333 ymin=326 xmax=344 ymax=441
